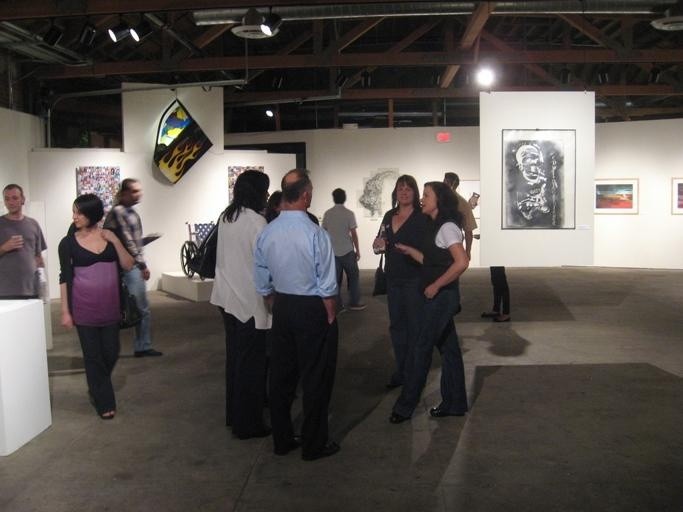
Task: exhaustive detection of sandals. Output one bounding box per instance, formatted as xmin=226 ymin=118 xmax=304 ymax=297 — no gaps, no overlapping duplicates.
xmin=481 ymin=312 xmax=500 ymax=317
xmin=99 ymin=407 xmax=115 ymax=420
xmin=494 ymin=316 xmax=510 ymax=322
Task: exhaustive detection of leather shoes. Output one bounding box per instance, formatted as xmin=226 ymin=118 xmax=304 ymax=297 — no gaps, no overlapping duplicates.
xmin=274 ymin=437 xmax=301 ymax=454
xmin=135 ymin=349 xmax=162 ymax=357
xmin=387 ymin=374 xmax=403 ymax=387
xmin=301 ymin=441 xmax=340 ymax=460
xmin=239 ymin=425 xmax=270 ymax=439
xmin=391 ymin=413 xmax=411 ymax=423
xmin=431 ymin=407 xmax=465 ymax=417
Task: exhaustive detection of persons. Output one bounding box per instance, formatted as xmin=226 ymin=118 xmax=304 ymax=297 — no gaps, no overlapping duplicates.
xmin=265 ymin=189 xmax=283 ymax=225
xmin=373 ymin=174 xmax=421 ymax=384
xmin=254 ymin=169 xmax=339 ymax=462
xmin=104 ymin=178 xmax=163 ymax=359
xmin=482 ymin=266 xmax=512 ymax=325
xmin=388 ymin=182 xmax=471 ymax=424
xmin=59 ymin=194 xmax=135 ymax=420
xmin=209 ymin=170 xmax=272 ymax=441
xmin=0 ymin=183 xmax=47 ymax=300
xmin=443 ymin=171 xmax=477 ymax=315
xmin=321 ymin=189 xmax=367 ymax=315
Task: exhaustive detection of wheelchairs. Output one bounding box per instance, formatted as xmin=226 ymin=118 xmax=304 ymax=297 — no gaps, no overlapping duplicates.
xmin=179 ymin=220 xmax=216 ymax=280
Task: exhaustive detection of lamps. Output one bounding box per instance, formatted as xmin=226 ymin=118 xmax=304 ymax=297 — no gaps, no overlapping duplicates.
xmin=129 ymin=20 xmax=155 ymax=42
xmin=43 ymin=20 xmax=66 ymax=46
xmin=260 ymin=7 xmax=282 ymax=37
xmin=154 ymin=90 xmax=213 ymax=184
xmin=266 ymin=104 xmax=279 ymax=117
xmin=79 ymin=18 xmax=97 ymax=46
xmin=107 ymin=23 xmax=135 ymax=42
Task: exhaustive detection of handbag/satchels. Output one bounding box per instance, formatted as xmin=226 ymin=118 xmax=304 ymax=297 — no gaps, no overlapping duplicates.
xmin=190 ymin=212 xmax=231 ymax=278
xmin=373 ymin=253 xmax=387 ymax=297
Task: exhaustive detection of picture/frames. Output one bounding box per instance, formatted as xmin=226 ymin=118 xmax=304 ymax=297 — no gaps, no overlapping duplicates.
xmin=228 ymin=166 xmax=265 ymax=202
xmin=500 ymin=129 xmax=576 ymax=229
xmin=456 ymin=180 xmax=481 ymax=219
xmin=591 ymin=177 xmax=640 ymax=214
xmin=75 ymin=166 xmax=121 ymax=221
xmin=671 ymin=179 xmax=683 ymax=215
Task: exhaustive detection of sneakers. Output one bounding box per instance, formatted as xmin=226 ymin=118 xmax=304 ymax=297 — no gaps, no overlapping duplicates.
xmin=350 ymin=304 xmax=367 ymax=310
xmin=337 ymin=305 xmax=347 ymax=313
